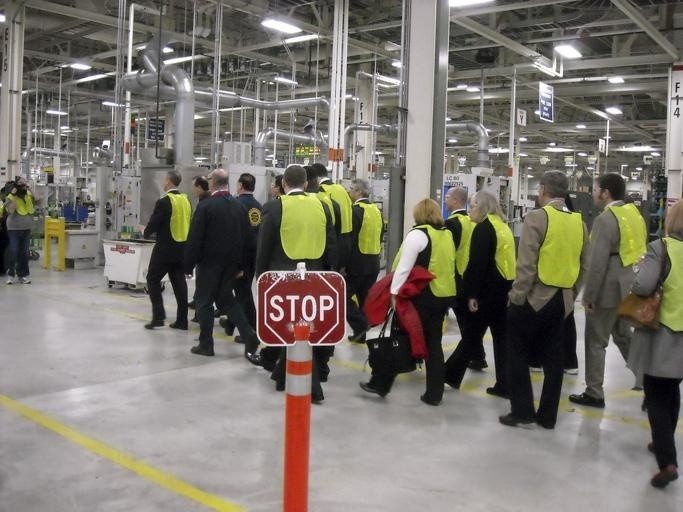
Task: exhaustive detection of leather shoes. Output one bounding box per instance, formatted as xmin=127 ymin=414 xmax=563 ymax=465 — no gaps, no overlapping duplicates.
xmin=570 ymin=390 xmax=607 ymax=408
xmin=143 ymin=309 xmax=334 ymax=401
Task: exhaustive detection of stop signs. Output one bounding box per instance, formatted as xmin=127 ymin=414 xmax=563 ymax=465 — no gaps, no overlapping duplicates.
xmin=257 ymin=269 xmax=346 ymax=347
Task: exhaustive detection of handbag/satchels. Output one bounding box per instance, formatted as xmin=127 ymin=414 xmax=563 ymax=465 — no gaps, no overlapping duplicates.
xmin=364 ymin=308 xmax=418 ymax=374
xmin=615 ymin=279 xmax=664 ymax=330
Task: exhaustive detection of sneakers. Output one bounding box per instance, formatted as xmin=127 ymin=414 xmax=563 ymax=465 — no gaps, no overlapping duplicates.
xmin=645 ymin=438 xmax=680 ymax=489
xmin=6 ymin=275 xmax=31 ymax=286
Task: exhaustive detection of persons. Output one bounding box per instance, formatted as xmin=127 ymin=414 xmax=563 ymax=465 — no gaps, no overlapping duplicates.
xmin=0 ymin=176 xmax=43 ymax=284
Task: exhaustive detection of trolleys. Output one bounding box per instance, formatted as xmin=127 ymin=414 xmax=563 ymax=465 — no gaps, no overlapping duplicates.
xmin=28 ymin=211 xmax=42 ymax=260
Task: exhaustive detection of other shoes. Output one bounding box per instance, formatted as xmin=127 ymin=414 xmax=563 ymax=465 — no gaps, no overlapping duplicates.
xmin=348 ymin=333 xmax=579 ymax=430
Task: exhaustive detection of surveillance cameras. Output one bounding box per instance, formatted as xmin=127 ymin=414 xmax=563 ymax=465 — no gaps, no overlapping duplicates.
xmin=102 ymin=140 xmax=110 ymax=150
